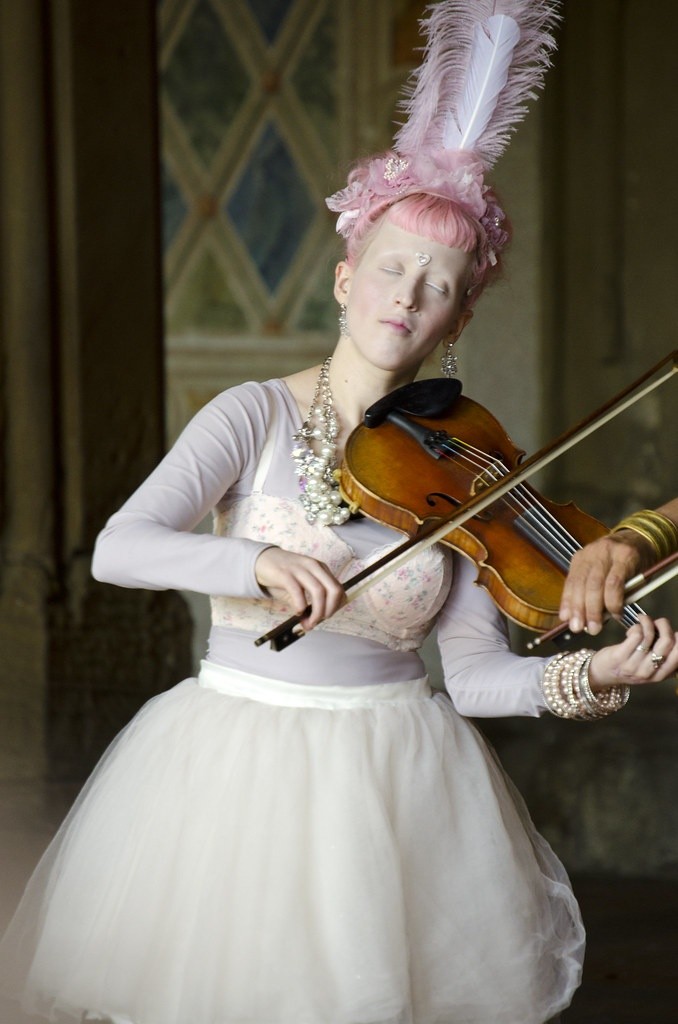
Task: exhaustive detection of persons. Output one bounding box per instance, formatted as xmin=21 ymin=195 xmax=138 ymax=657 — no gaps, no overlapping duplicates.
xmin=16 ymin=192 xmax=678 ymax=1024
xmin=559 ymin=479 xmax=678 ymax=634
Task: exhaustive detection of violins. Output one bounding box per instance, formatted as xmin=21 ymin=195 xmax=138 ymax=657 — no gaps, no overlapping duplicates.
xmin=340 ymin=376 xmax=652 ymax=636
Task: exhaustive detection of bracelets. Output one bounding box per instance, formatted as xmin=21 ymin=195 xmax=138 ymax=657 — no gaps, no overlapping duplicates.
xmin=607 ymin=505 xmax=677 ymax=562
xmin=540 ymin=648 xmax=630 ymax=722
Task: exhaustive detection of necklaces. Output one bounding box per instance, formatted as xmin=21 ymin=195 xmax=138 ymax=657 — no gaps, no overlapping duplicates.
xmin=295 ymin=355 xmax=375 ymax=528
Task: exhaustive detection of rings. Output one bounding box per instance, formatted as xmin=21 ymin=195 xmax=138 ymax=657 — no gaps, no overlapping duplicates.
xmin=635 ymin=643 xmax=649 ymax=654
xmin=650 ymin=652 xmax=667 ymax=669
